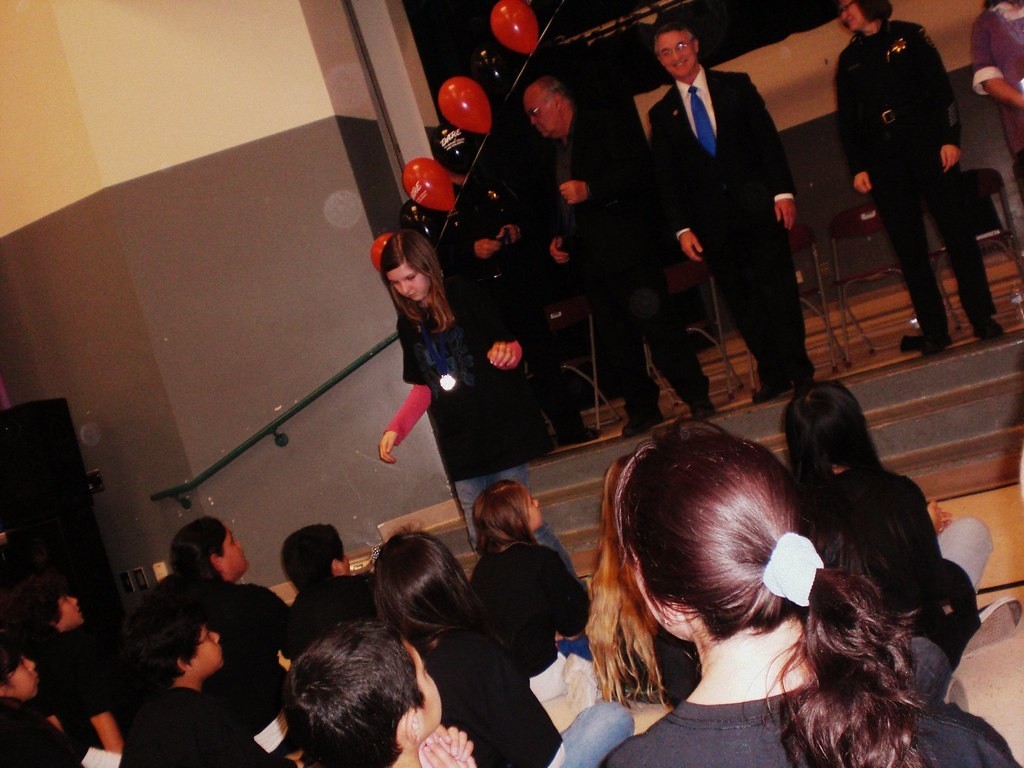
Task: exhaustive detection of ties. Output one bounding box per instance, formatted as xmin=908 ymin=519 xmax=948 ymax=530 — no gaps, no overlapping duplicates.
xmin=688 ymin=87 xmax=717 ymax=156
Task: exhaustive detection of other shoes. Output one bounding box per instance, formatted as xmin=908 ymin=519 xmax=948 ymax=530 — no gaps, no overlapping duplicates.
xmin=753 ymin=376 xmax=783 ymax=401
xmin=621 ymin=407 xmax=664 ymax=438
xmin=690 ymin=397 xmax=715 ymax=419
xmin=921 ymin=333 xmax=951 ymax=354
xmin=972 ymin=318 xmax=1004 ymax=338
xmin=557 ymin=428 xmax=597 ymax=445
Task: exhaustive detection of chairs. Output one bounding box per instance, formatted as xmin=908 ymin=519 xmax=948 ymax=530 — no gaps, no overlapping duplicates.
xmin=643 ymin=260 xmax=747 ymax=410
xmin=748 ymin=226 xmax=844 ymax=394
xmin=520 ymin=289 xmax=624 ymax=439
xmin=933 ymin=167 xmax=1024 ymax=333
xmin=829 ymin=207 xmax=964 ymax=369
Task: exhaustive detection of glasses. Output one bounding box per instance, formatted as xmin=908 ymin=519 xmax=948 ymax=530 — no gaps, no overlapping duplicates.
xmin=659 ymin=36 xmax=695 ymax=61
xmin=528 ymin=98 xmax=552 ymax=116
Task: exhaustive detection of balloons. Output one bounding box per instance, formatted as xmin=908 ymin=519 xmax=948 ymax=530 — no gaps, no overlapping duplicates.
xmin=438 ymin=76 xmax=492 ymax=135
xmin=403 ymin=158 xmax=455 ymax=212
xmin=490 ymin=0 xmax=539 ymax=53
xmin=370 ymin=232 xmax=394 ymax=270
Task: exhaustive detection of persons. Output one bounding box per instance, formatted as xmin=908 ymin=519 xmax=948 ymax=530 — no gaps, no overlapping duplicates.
xmin=379 ymin=231 xmax=581 ymax=582
xmin=0 ymin=452 xmax=701 ymax=768
xmin=414 ymin=23 xmax=815 ymax=446
xmin=834 ymin=0 xmax=1005 ymax=355
xmin=601 ymin=418 xmax=1023 ymax=768
xmin=782 ymin=380 xmax=1022 ymax=669
xmin=973 ymin=0 xmax=1024 ymax=213
